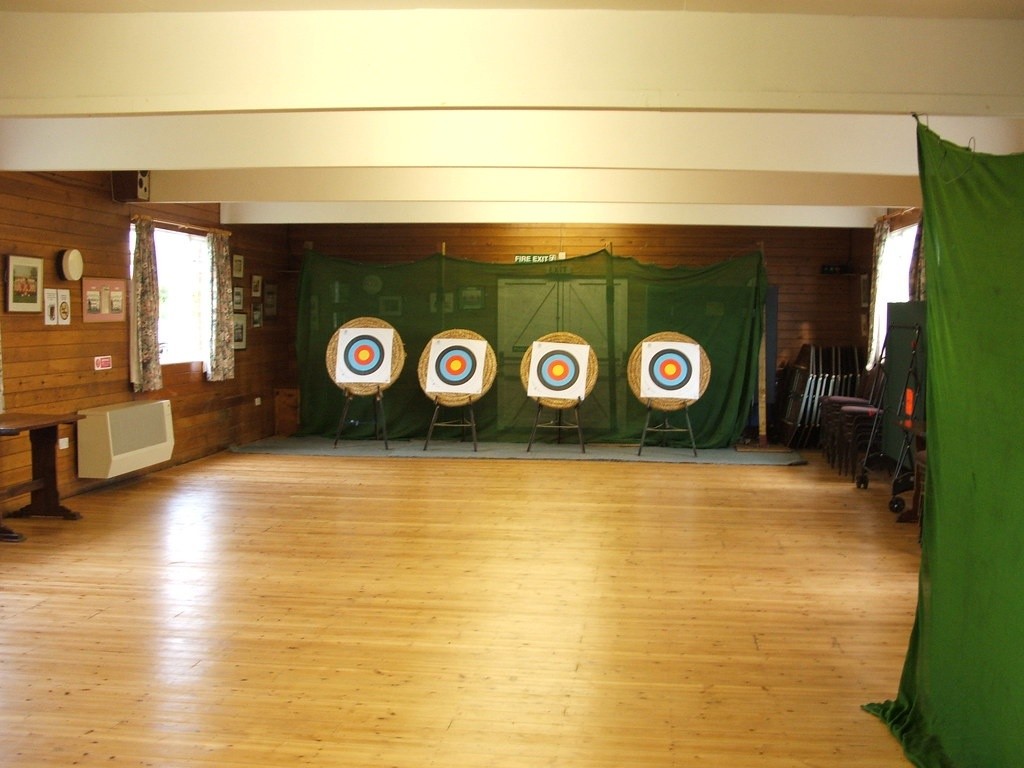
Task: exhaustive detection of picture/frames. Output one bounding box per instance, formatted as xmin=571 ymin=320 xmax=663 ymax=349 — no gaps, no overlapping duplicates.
xmin=233 ymin=313 xmax=247 ymax=350
xmin=262 ymin=279 xmax=280 ymax=321
xmin=250 ymin=302 xmax=264 ymax=329
xmin=5 ymin=254 xmax=44 ymax=313
xmin=81 ymin=276 xmax=128 ymax=323
xmin=250 ymin=274 xmax=263 ymax=297
xmin=232 ymin=254 xmax=244 ymax=278
xmin=232 ymin=286 xmax=244 ymax=311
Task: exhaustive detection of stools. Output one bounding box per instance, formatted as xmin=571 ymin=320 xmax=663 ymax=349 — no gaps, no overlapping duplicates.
xmin=912 ymin=451 xmax=928 ymax=549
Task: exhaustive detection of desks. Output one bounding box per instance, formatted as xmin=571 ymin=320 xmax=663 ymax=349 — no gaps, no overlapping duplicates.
xmin=856 ymin=302 xmax=929 ymax=513
xmin=896 ymin=420 xmax=927 ymax=520
xmin=0 ymin=413 xmax=86 ymax=544
xmin=778 ymin=343 xmax=859 ymax=451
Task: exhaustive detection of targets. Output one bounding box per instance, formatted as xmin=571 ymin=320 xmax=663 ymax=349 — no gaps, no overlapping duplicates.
xmin=525 ymin=341 xmax=590 ymax=403
xmin=333 ymin=325 xmax=395 ymax=384
xmin=640 ymin=341 xmax=702 ymax=402
xmin=427 ymin=338 xmax=486 ymax=396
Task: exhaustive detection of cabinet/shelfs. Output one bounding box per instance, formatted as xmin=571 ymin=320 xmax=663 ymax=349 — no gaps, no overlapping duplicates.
xmin=77 ymin=400 xmax=177 ymax=479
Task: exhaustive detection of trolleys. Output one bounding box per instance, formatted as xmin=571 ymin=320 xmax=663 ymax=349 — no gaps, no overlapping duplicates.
xmin=854 ymin=301 xmax=929 ymax=513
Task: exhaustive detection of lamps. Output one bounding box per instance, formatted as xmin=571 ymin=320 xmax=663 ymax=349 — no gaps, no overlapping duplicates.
xmin=56 ymin=249 xmax=84 ymax=282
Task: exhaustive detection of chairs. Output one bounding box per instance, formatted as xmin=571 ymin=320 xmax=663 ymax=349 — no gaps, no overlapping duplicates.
xmin=817 ymin=358 xmax=892 ymax=484
